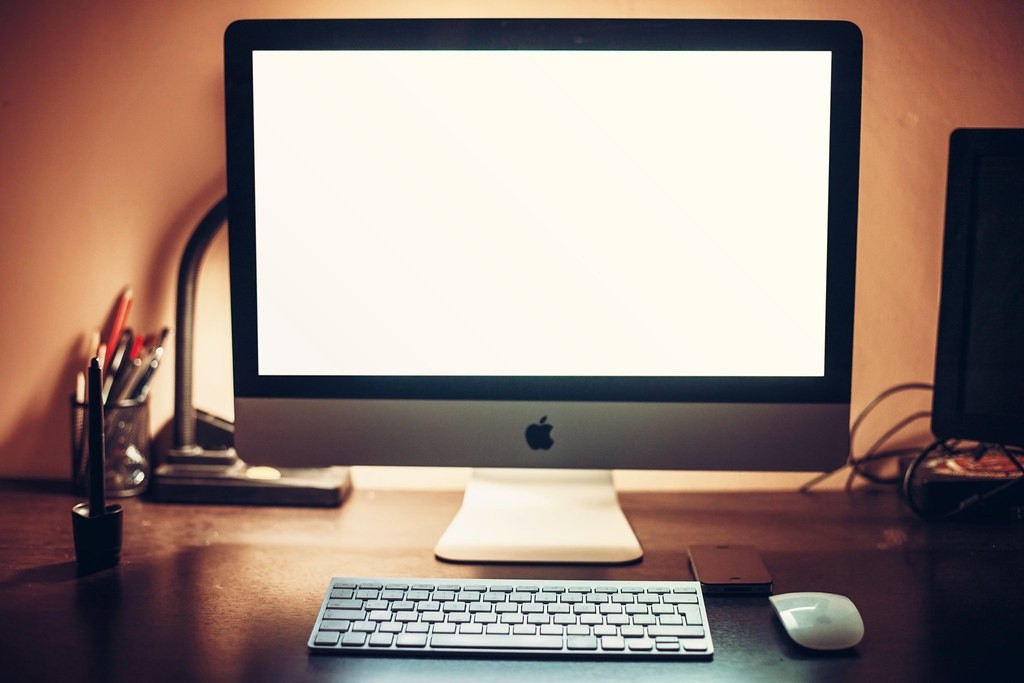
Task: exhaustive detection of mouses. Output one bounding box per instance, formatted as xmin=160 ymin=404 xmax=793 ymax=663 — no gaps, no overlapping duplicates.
xmin=768 ymin=592 xmax=864 ymax=650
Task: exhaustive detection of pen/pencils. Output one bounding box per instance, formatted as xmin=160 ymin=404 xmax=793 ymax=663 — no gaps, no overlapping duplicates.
xmin=75 ymin=286 xmax=170 ymax=473
xmin=85 ymin=358 xmax=106 ymax=518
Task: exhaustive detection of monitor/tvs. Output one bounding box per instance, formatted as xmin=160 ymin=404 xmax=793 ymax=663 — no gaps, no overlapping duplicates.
xmin=223 ymin=18 xmax=864 ymax=563
xmin=931 ymin=125 xmax=1024 ymax=451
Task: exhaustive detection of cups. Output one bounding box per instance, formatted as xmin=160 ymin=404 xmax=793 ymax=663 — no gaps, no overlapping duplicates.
xmin=72 ymin=396 xmax=148 ymax=500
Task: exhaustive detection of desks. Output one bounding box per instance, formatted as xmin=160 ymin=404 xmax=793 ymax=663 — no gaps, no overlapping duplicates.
xmin=0 ymin=476 xmax=1024 ymax=683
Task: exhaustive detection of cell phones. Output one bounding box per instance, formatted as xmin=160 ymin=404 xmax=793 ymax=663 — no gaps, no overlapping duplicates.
xmin=686 ymin=540 xmax=773 ymax=595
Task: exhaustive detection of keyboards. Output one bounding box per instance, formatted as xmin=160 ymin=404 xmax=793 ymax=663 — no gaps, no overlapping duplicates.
xmin=309 ymin=578 xmax=714 ymax=660
